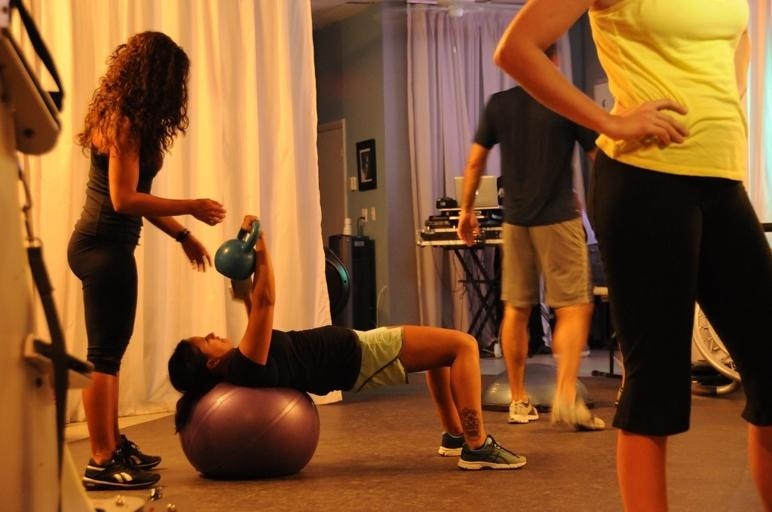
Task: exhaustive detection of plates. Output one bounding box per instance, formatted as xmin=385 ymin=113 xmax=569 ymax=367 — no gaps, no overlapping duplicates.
xmin=417 ymin=239 xmax=534 ymax=358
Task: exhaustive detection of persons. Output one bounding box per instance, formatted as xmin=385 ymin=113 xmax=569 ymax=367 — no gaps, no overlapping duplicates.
xmin=66 ymin=31 xmax=226 ymax=492
xmin=458 ymin=42 xmax=606 ymax=431
xmin=493 ymin=0 xmax=772 ymax=510
xmin=169 ymin=215 xmax=527 ymax=472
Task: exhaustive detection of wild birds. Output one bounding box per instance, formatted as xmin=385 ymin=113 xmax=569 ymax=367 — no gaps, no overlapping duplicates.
xmin=455 ymin=175 xmax=498 ymax=207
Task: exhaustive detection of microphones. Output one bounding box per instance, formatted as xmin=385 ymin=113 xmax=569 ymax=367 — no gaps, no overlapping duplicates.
xmin=589 ymin=286 xmax=623 ymax=378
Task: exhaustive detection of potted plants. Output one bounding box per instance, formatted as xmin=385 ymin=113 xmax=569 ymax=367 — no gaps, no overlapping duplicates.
xmin=355 ymin=137 xmax=375 ymax=190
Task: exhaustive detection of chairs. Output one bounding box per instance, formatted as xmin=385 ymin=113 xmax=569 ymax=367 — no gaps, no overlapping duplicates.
xmin=438 ymin=432 xmax=465 ymax=457
xmin=552 ymin=397 xmax=606 ymax=430
xmin=456 ymin=437 xmax=527 ymax=470
xmin=116 ymin=434 xmax=161 ymax=471
xmin=81 ymin=454 xmax=162 ymax=491
xmin=507 ymin=399 xmax=540 ymax=424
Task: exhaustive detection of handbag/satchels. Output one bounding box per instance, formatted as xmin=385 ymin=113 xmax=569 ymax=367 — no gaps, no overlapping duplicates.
xmin=176 ymin=228 xmax=190 ymax=242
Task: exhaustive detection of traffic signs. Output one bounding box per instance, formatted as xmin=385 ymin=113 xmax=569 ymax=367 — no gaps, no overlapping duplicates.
xmin=360 ymin=208 xmax=368 ymax=222
